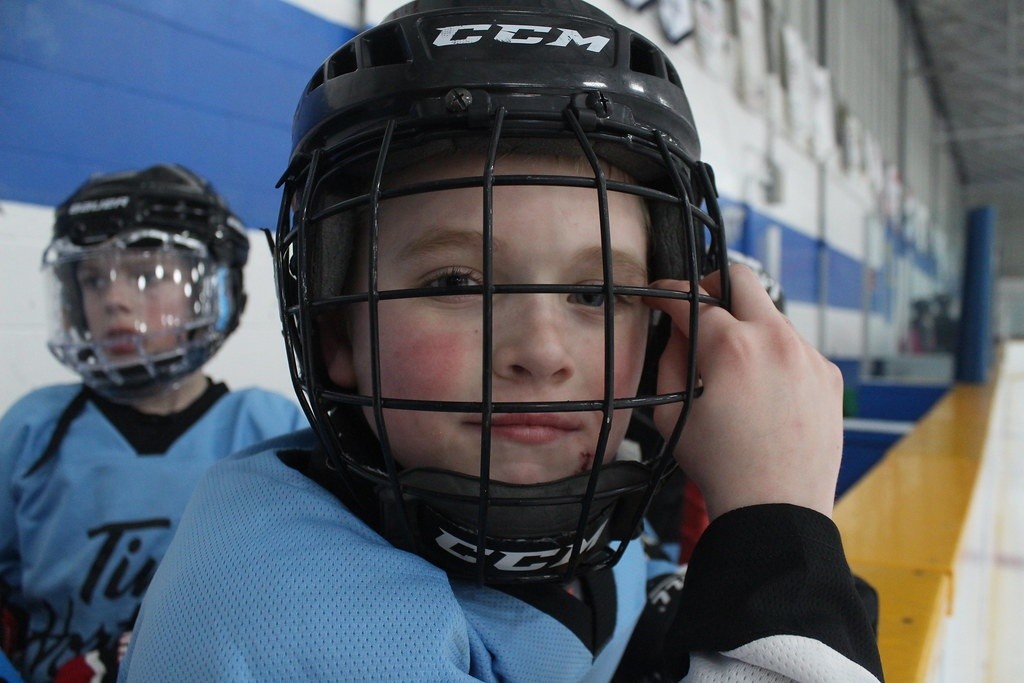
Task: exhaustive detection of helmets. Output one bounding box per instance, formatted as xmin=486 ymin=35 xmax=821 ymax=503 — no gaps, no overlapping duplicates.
xmin=46 ymin=162 xmax=250 ymax=401
xmin=264 ymin=1 xmax=733 ymax=582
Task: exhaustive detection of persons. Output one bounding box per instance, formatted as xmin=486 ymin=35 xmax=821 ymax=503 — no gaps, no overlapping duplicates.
xmin=112 ymin=1 xmax=884 ymax=683
xmin=907 ymin=291 xmax=955 ymax=356
xmin=0 ymin=162 xmax=313 ymax=683
